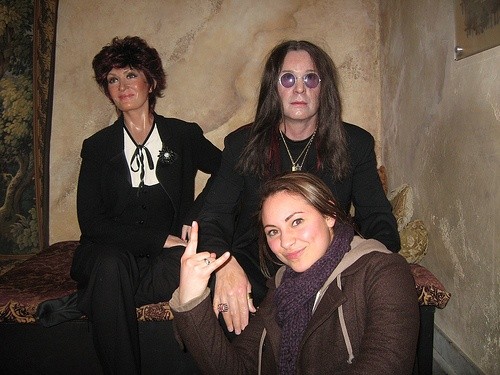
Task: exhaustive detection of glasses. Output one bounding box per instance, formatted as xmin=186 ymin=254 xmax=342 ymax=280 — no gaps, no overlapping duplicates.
xmin=278 ymin=73 xmax=322 ymax=88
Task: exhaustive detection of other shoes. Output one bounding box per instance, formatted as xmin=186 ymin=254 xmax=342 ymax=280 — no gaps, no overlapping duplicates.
xmin=37 ymin=292 xmax=84 ymax=327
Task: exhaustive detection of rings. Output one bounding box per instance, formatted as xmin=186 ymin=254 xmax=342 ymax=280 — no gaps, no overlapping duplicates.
xmin=218 ymin=304 xmax=228 ymax=311
xmin=247 ymin=292 xmax=252 ymax=300
xmin=204 ymin=258 xmax=210 ymax=264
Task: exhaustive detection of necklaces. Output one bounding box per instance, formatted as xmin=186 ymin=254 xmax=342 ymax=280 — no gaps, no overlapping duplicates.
xmin=279 ymin=122 xmax=317 ymax=173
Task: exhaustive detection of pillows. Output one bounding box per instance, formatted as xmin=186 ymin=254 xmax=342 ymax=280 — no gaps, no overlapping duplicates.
xmin=377 ymin=165 xmax=430 ymax=264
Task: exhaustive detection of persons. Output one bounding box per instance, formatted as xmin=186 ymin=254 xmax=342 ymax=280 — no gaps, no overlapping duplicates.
xmin=189 ymin=40 xmax=402 ymax=343
xmin=170 ymin=171 xmax=420 ymax=375
xmin=67 ymin=34 xmax=224 ymax=375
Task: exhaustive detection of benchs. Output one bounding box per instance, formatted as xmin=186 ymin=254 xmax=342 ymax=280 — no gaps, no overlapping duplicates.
xmin=1 ymin=241 xmax=452 ymax=375
